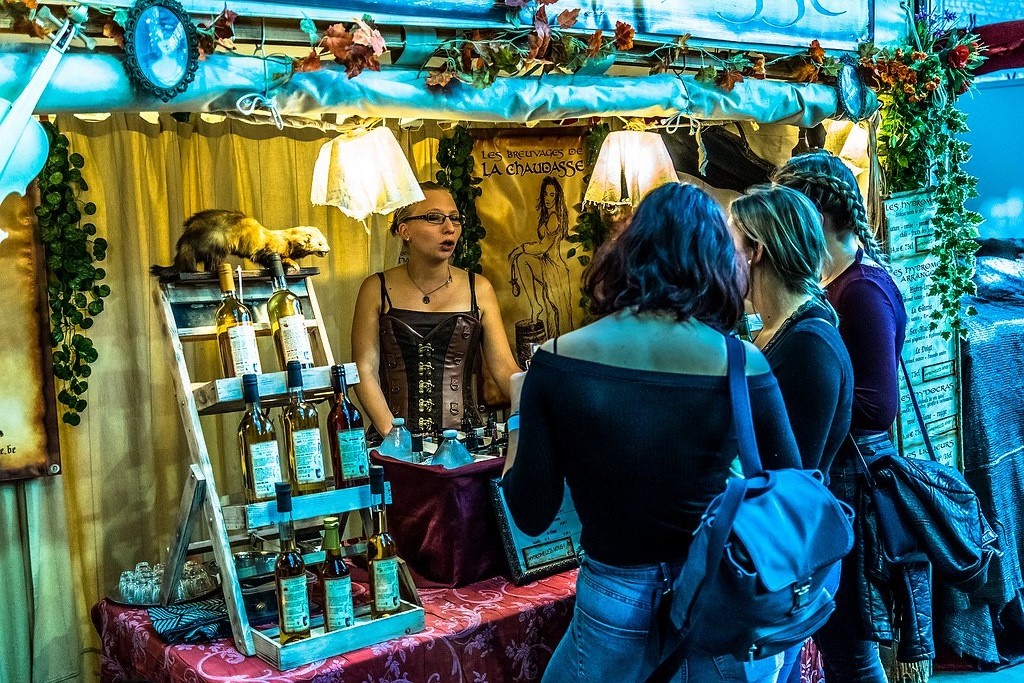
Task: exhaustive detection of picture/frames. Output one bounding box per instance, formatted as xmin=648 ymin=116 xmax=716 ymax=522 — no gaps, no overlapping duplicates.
xmin=489 ymin=473 xmax=586 ymax=588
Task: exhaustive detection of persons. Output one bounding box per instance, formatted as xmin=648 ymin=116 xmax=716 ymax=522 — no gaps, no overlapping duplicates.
xmin=349 ymin=178 xmax=526 ymax=449
xmin=499 ymin=179 xmax=812 ymax=683
xmin=726 ymin=184 xmax=857 ymax=683
xmin=776 ymin=152 xmax=931 ymax=682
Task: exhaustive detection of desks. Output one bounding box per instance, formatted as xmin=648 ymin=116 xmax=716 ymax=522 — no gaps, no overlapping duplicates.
xmin=90 ymin=558 xmax=580 ymax=683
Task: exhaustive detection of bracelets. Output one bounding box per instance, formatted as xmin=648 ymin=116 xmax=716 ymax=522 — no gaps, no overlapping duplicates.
xmin=506 ymin=411 xmax=521 ymax=433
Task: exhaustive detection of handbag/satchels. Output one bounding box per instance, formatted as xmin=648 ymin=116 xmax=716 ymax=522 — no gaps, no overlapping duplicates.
xmin=871 ymin=485 xmax=927 ymax=560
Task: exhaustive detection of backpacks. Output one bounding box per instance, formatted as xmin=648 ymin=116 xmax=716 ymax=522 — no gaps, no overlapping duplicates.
xmin=650 ymin=334 xmax=854 ymax=681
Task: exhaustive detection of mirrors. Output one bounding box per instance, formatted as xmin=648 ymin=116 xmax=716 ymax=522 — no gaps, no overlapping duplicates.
xmin=121 ymin=1 xmax=199 ymax=100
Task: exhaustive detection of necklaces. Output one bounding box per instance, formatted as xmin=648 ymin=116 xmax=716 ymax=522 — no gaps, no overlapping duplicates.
xmin=405 ymin=256 xmax=454 ymax=306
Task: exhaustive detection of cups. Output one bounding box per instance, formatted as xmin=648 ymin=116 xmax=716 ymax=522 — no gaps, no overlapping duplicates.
xmin=118 ymin=560 xmax=215 ymax=604
xmin=230 ymin=550 xmax=279 ymax=578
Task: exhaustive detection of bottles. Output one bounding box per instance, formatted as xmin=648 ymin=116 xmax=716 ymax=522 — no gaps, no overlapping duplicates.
xmin=214 ymin=263 xmax=261 ymax=379
xmin=236 ymin=373 xmax=282 ymax=505
xmin=462 ymin=414 xmax=509 ymax=458
xmin=319 ymin=516 xmax=358 ymax=634
xmin=379 ymin=418 xmax=422 ymax=463
xmin=281 ymin=360 xmax=328 ymax=497
xmin=274 ymin=482 xmax=312 ymax=645
xmin=431 ymin=429 xmax=475 ymax=470
xmin=368 ymin=466 xmax=400 ymax=621
xmin=327 ymin=363 xmax=372 ymax=490
xmin=266 ymin=252 xmax=315 ymax=372
xmin=410 ymin=430 xmax=425 ymax=464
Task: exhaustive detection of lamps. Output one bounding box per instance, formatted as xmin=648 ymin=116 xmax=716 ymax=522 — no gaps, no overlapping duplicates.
xmin=311 ymin=125 xmax=423 ymax=223
xmin=583 ymin=129 xmax=679 ymax=207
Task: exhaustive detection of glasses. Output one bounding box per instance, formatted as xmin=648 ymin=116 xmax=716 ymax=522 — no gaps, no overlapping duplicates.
xmin=404 ymin=212 xmax=466 ymax=225
